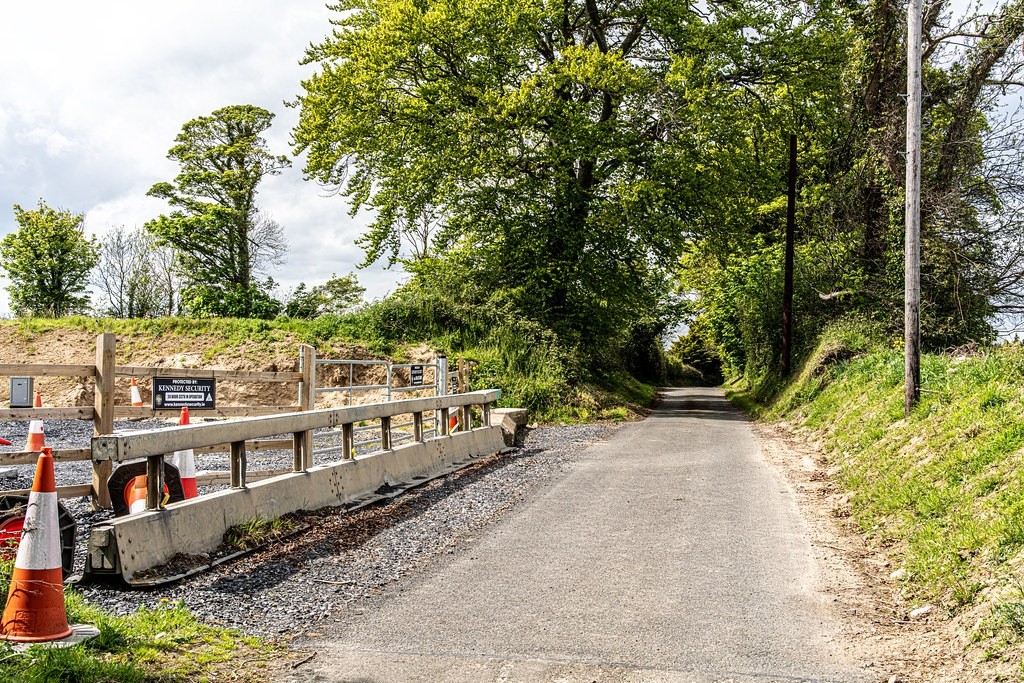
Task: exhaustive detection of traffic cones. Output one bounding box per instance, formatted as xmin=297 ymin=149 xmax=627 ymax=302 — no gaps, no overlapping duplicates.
xmin=0 ymin=437 xmax=12 ymax=445
xmin=171 ymin=405 xmax=198 ymax=500
xmin=106 ymin=458 xmax=185 ymax=519
xmin=129 ymin=377 xmax=142 ymax=406
xmin=447 ymin=415 xmax=459 ymax=433
xmin=0 ymin=494 xmax=77 ymax=582
xmin=0 ymin=447 xmax=72 ymax=641
xmin=24 ymin=390 xmax=45 ymax=451
